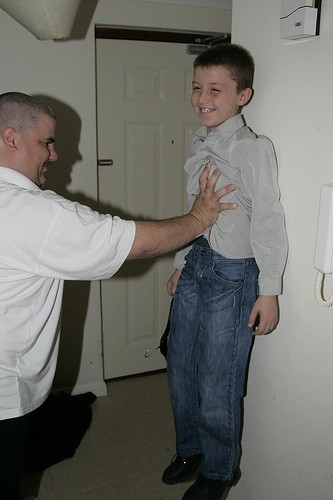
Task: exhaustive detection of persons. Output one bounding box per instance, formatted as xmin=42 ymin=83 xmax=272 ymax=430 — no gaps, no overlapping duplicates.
xmin=0 ymin=91 xmax=238 ymax=500
xmin=162 ymin=44 xmax=289 ymax=500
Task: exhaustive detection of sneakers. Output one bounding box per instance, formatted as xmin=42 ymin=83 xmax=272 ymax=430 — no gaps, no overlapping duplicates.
xmin=162 ymin=453 xmax=204 ymax=485
xmin=181 ymin=467 xmax=241 ymax=500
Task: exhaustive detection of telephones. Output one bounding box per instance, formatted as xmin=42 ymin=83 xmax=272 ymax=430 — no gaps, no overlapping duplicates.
xmin=313 ymin=181 xmax=333 ymax=308
